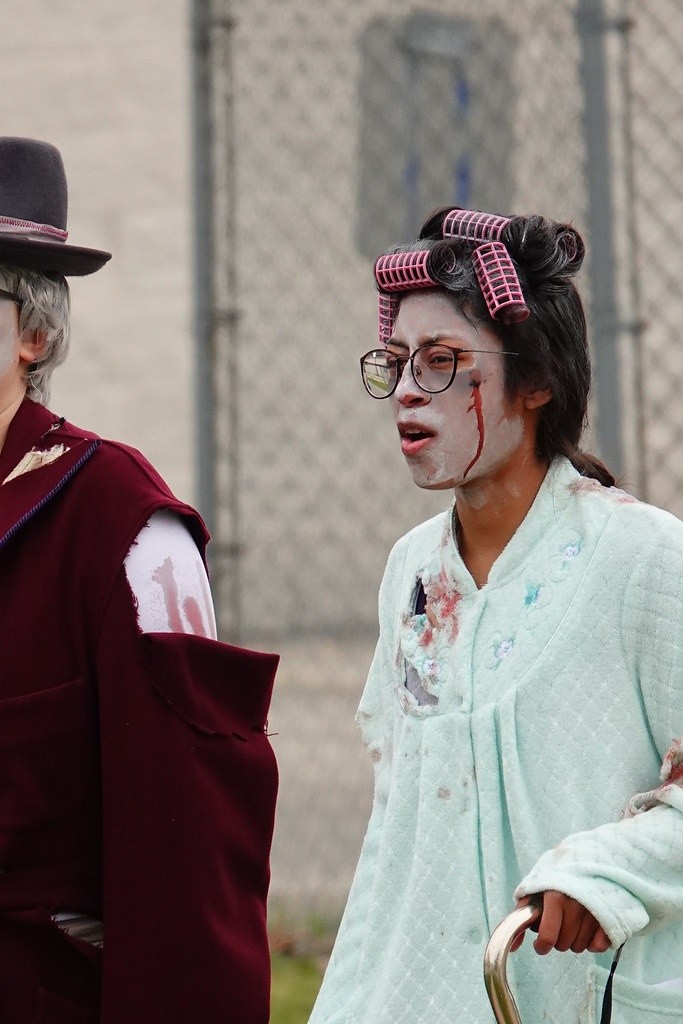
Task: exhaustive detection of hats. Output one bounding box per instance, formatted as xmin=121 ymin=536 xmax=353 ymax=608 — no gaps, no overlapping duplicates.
xmin=0 ymin=137 xmax=112 ymax=276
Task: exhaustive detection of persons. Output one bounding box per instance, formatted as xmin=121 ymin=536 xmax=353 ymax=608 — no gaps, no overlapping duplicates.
xmin=0 ymin=136 xmax=279 ymax=1024
xmin=305 ymin=208 xmax=683 ymax=1024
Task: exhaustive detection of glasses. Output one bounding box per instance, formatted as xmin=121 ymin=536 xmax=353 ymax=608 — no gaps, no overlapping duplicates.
xmin=360 ymin=343 xmax=522 ymax=399
